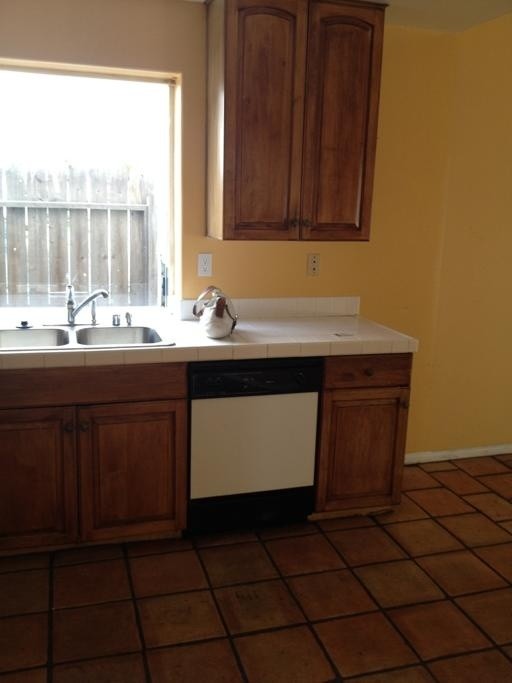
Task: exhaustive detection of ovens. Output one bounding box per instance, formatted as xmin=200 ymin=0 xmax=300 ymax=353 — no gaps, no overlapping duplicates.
xmin=188 ymin=363 xmax=323 ymax=534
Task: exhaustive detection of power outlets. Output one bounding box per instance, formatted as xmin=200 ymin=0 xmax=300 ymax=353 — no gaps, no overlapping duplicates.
xmin=198 ymin=254 xmax=213 ymax=277
xmin=307 ymin=253 xmax=320 ymax=277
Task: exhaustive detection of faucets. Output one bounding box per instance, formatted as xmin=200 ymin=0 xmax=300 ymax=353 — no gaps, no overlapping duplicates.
xmin=65 ymin=284 xmax=111 ymax=327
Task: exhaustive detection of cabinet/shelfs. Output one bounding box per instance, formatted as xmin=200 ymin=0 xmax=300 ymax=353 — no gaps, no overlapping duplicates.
xmin=202 ymin=0 xmax=387 ymax=243
xmin=1 ymin=396 xmax=190 ymax=555
xmin=316 ymin=353 xmax=414 ymax=517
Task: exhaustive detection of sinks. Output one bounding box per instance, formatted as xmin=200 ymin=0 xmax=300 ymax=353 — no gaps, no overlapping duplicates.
xmin=0 ymin=329 xmax=71 ymax=349
xmin=75 ymin=326 xmax=162 ymax=346
